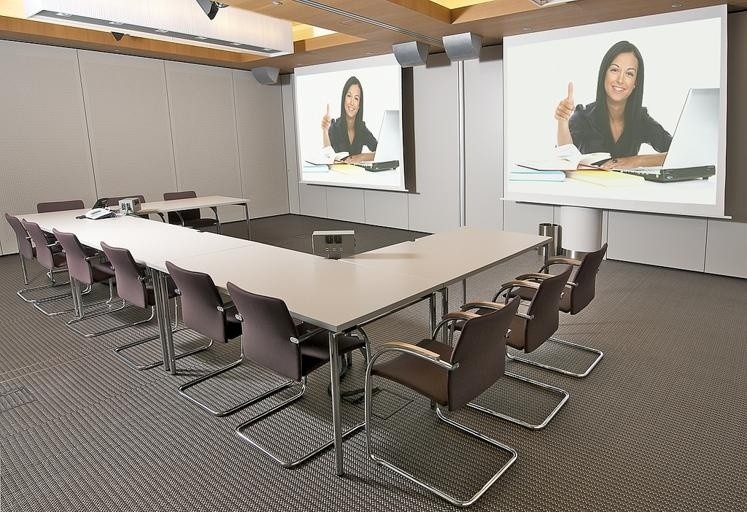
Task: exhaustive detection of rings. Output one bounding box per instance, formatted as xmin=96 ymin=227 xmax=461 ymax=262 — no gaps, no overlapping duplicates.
xmin=610 ymin=158 xmax=617 ymax=164
xmin=349 ymin=157 xmax=352 ymax=158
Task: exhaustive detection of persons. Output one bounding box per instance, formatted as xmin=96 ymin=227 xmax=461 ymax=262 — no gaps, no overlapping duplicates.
xmin=320 ymin=76 xmax=378 ymax=165
xmin=553 ymin=39 xmax=673 ymax=171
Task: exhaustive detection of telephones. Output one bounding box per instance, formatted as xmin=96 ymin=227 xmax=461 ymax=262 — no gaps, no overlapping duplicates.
xmin=84 ymin=207 xmax=117 ymax=220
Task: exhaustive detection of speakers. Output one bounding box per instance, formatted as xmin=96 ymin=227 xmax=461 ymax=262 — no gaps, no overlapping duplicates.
xmin=392 ymin=42 xmax=431 ymax=69
xmin=251 ymin=67 xmax=280 ymax=86
xmin=442 ymin=33 xmax=484 ymax=62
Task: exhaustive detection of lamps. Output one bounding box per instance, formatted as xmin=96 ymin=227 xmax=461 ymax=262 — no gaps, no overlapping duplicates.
xmin=196 ymin=0 xmax=219 ymax=21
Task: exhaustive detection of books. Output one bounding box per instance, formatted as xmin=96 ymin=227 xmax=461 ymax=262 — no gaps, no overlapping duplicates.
xmin=507 ymin=144 xmax=646 ymax=191
xmin=303 ymin=145 xmax=366 ymax=176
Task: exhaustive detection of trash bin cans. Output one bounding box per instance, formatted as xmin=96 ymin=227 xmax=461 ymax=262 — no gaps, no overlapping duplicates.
xmin=538 ymin=223 xmax=558 ymax=257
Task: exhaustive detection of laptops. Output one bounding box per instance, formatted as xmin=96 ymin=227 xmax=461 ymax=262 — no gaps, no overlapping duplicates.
xmin=612 ymin=88 xmax=719 ymax=183
xmin=354 ymin=110 xmax=404 ymax=173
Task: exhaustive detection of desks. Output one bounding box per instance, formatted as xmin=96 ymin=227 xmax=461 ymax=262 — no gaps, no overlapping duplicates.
xmin=105 ymin=195 xmax=251 ymax=241
xmin=338 ymin=224 xmax=554 ymax=346
xmin=11 ymin=208 xmax=444 ymax=478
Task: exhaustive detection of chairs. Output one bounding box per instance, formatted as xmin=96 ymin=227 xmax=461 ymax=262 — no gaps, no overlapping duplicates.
xmin=52 ymin=227 xmax=156 ymax=338
xmin=447 ymin=263 xmax=573 ymax=432
xmin=36 ymin=191 xmax=220 ymax=234
xmin=21 ymin=218 xmax=126 ymax=317
xmin=99 ymin=240 xmax=214 ymax=371
xmin=226 ymin=281 xmax=371 ymax=469
xmin=4 ymin=212 xmax=90 ymax=303
xmin=165 ymin=260 xmax=294 ymax=417
xmin=364 ymin=294 xmax=521 ymax=508
xmin=502 ymin=241 xmax=609 ymax=378
